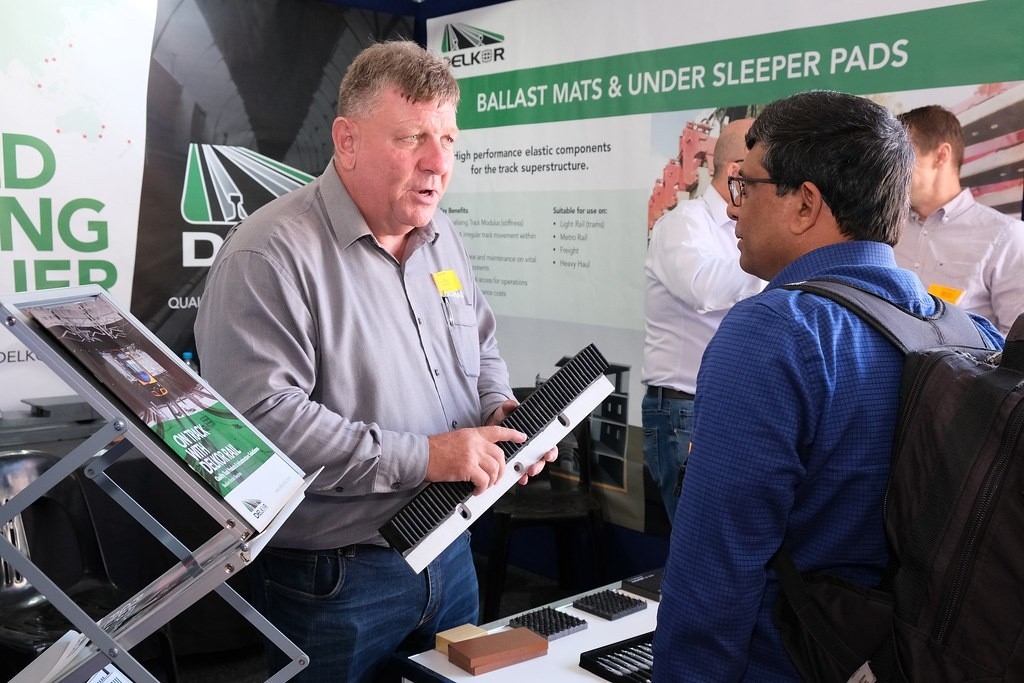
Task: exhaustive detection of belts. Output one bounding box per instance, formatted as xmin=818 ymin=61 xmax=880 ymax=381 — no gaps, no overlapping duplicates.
xmin=645 ymin=385 xmax=695 ymax=401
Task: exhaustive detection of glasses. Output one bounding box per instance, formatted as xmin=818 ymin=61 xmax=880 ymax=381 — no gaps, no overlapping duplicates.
xmin=727 ymin=175 xmax=834 ymax=215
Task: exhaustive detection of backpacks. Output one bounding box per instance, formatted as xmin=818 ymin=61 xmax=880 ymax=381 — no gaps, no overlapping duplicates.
xmin=769 ymin=280 xmax=1024 ymax=683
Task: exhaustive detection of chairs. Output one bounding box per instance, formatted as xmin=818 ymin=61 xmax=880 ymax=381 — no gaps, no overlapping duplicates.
xmin=73 ymin=458 xmax=267 ymax=659
xmin=0 ymin=447 xmax=180 ymax=683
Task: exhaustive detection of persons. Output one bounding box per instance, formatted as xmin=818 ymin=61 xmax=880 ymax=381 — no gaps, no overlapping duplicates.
xmin=193 ymin=40 xmax=559 ymax=682
xmin=639 ymin=117 xmax=773 ymax=533
xmin=650 ymin=90 xmax=1007 ymax=683
xmin=888 ymin=104 xmax=1024 ymax=343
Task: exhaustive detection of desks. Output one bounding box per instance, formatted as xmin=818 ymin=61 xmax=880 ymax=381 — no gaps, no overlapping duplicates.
xmin=392 ymin=567 xmax=663 ymax=683
